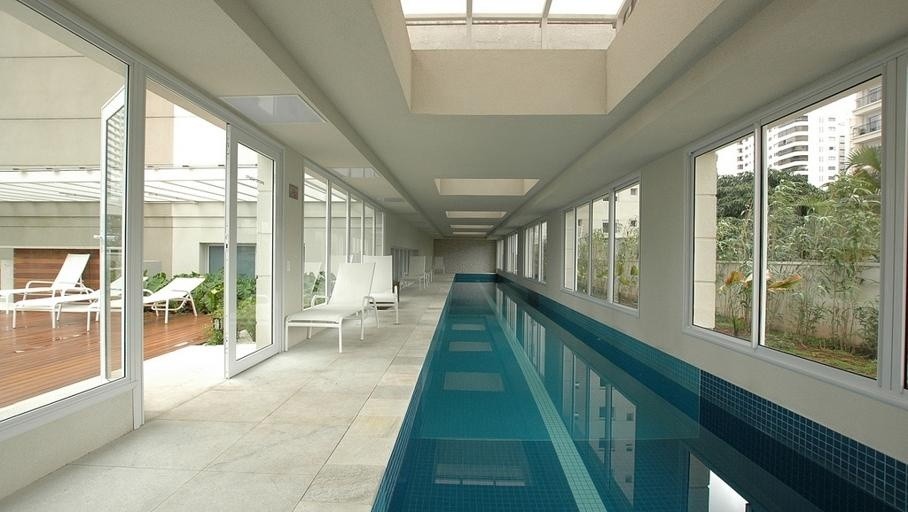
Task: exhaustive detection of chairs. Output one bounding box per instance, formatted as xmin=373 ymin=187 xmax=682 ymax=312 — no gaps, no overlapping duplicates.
xmin=434 ymin=256 xmax=445 ymax=273
xmin=399 ymin=255 xmax=433 ymax=288
xmin=0 ymin=253 xmax=207 ymax=328
xmin=283 ymin=253 xmax=401 ymax=354
